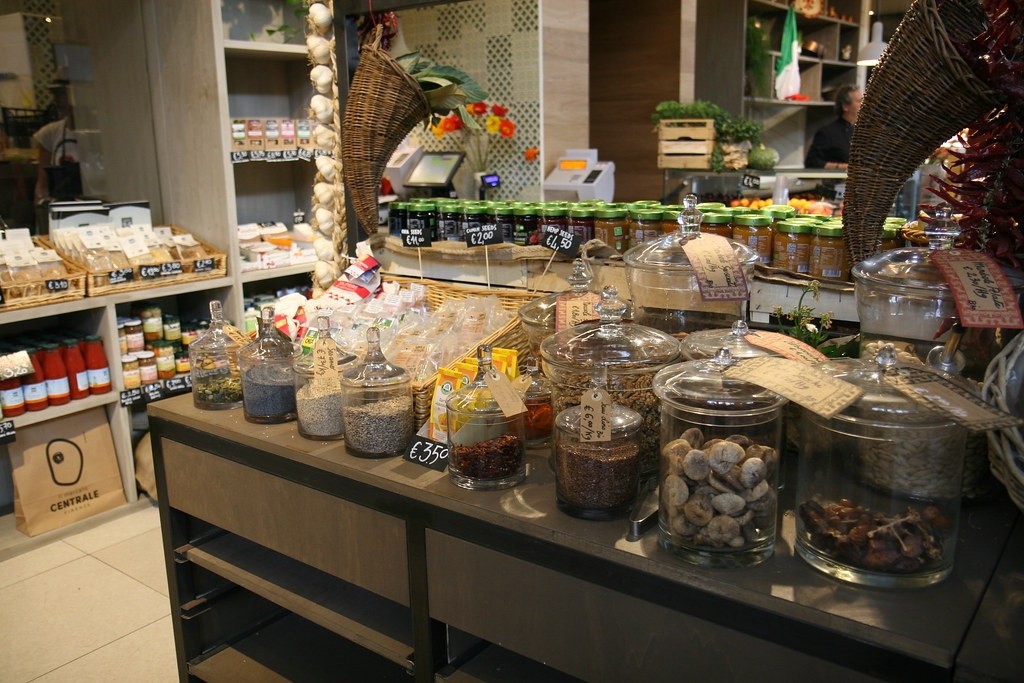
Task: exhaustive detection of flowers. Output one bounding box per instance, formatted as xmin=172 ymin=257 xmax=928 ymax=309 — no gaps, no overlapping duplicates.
xmin=433 ymin=104 xmax=540 ymax=173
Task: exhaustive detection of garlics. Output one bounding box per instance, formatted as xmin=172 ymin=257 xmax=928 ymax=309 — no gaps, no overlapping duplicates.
xmin=306 ymin=2 xmax=339 ymax=301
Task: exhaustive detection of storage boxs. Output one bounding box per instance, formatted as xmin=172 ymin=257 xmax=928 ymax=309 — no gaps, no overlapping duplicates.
xmin=658 ymin=118 xmax=715 ymax=141
xmin=657 ymin=154 xmax=711 ymax=169
xmin=232 ymin=116 xmax=315 ymax=151
xmin=657 ymin=140 xmax=716 ymax=154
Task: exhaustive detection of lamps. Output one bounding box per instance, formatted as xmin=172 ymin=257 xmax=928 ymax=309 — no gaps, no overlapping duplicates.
xmin=856 ymin=0 xmax=889 ymax=66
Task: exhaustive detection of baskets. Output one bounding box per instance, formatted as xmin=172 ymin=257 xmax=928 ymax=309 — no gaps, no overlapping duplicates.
xmin=983 ymin=330 xmax=1024 ymax=506
xmin=223 ymin=272 xmax=549 ymax=432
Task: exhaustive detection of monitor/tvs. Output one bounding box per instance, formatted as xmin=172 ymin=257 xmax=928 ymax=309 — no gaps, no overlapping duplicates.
xmin=403 ymin=151 xmax=466 ymax=188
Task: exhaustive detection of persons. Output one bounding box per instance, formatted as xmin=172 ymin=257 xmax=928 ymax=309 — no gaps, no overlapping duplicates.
xmin=804 ymin=84 xmax=863 ymax=194
xmin=34 ymin=70 xmax=79 ymax=197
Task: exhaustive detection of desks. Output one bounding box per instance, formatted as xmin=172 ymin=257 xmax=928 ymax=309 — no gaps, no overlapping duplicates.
xmin=526 ymin=256 xmax=742 ymax=319
xmin=752 ymin=281 xmax=970 ymax=335
xmin=370 ymin=244 xmax=526 ymax=288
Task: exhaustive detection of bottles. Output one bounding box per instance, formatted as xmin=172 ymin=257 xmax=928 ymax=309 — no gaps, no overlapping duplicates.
xmin=512 ymin=356 xmax=551 ymax=445
xmin=445 ymin=344 xmax=528 ymax=492
xmin=652 ymin=348 xmax=789 ymax=569
xmin=555 ymin=379 xmax=643 ymax=521
xmin=540 ymin=285 xmax=680 ymax=474
xmin=340 ymin=326 xmax=414 ymax=458
xmin=388 ymin=198 xmax=850 ymax=356
xmin=0 ymin=328 xmax=112 ymax=417
xmin=116 ymin=303 xmax=230 ymax=389
xmin=680 ymin=319 xmax=783 ymax=360
xmin=293 ymin=317 xmax=363 ymax=440
xmin=794 ymin=344 xmax=967 ymax=587
xmin=188 ymin=300 xmax=252 ymax=409
xmin=236 ymin=307 xmax=304 ymax=424
xmin=851 ymin=207 xmax=985 ymax=502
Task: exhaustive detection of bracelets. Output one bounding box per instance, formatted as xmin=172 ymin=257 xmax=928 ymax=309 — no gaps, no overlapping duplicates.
xmin=836 ymin=164 xmax=839 ymax=168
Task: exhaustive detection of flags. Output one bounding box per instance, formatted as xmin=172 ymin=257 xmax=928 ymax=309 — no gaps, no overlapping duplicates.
xmin=774 ymin=7 xmax=809 ymax=101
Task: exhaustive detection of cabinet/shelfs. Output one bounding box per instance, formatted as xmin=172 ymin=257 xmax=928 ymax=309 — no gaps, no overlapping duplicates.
xmin=146 ymin=258 xmax=1014 ymax=683
xmin=0 ymin=0 xmax=341 ymax=558
xmin=698 ymin=0 xmax=859 ymax=171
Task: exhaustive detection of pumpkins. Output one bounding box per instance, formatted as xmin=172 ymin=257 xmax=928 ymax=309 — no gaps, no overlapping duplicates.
xmin=748 ymin=142 xmax=779 ymax=170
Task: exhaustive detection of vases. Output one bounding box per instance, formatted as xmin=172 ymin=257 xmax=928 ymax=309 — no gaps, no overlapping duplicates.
xmin=471 ymin=172 xmax=489 ymax=198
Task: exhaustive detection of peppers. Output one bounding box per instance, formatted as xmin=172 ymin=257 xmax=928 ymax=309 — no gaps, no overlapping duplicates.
xmin=918 ymin=0 xmax=1024 ymax=339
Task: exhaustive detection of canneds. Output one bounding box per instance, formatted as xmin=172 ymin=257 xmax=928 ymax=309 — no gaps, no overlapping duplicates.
xmin=387 ymin=196 xmax=909 ymax=282
xmin=117 ymin=302 xmax=233 ymax=390
